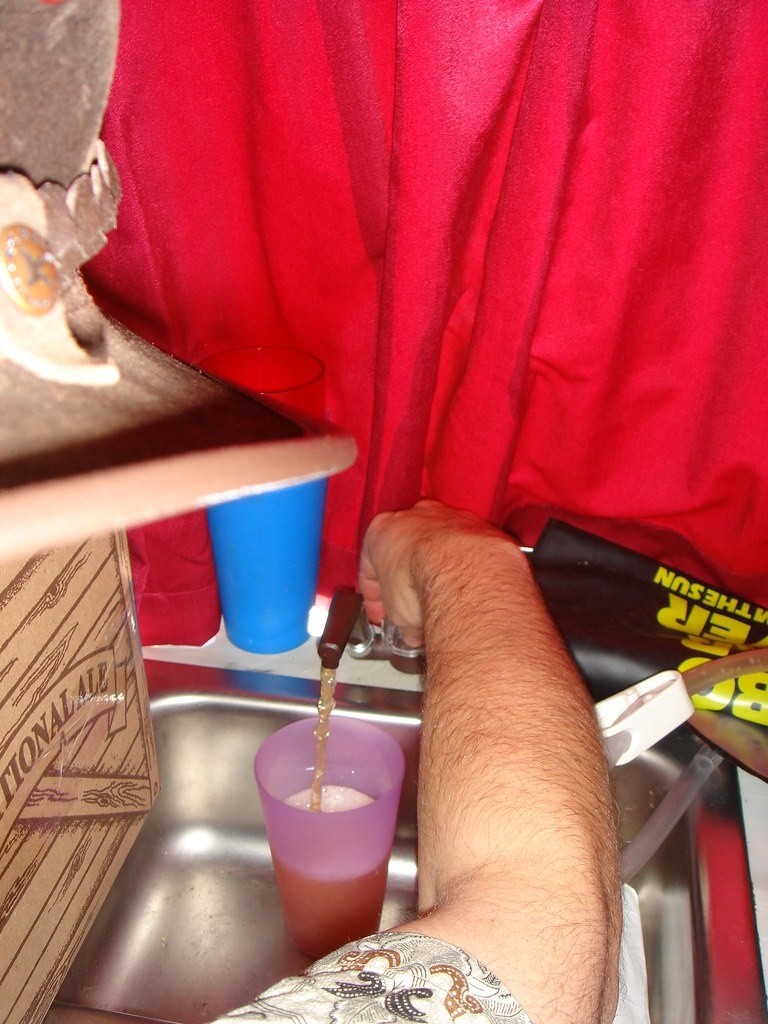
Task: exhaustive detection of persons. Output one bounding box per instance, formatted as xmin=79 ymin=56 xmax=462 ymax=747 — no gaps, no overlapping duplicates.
xmin=213 ymin=499 xmax=623 ymax=1024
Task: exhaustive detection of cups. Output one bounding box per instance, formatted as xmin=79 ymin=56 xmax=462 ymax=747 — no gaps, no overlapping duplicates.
xmin=254 ymin=715 xmax=405 ymax=957
xmin=200 ymin=345 xmax=327 ymax=436
xmin=206 ymin=478 xmax=329 ymax=654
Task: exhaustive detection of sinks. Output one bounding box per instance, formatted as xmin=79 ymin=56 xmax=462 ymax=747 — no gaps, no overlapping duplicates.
xmin=41 ymin=657 xmax=768 ymax=1024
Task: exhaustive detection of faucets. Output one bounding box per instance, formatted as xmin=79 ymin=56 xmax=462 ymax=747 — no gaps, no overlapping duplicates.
xmin=317 ymin=585 xmax=376 ymax=669
